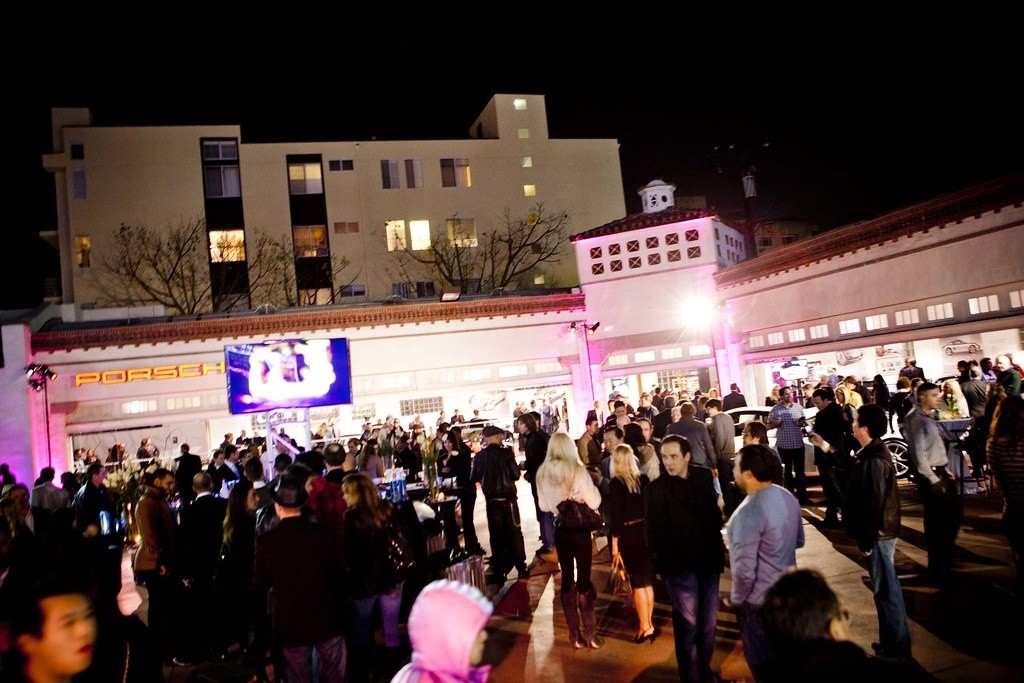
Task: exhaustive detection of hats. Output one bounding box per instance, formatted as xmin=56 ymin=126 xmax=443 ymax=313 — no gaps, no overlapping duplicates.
xmin=270 ymin=474 xmax=308 ymax=507
xmin=483 ymin=426 xmax=504 ymax=436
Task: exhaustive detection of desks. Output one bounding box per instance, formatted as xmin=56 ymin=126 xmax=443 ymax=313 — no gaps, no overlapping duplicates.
xmin=372 ymin=475 xmax=461 ymax=557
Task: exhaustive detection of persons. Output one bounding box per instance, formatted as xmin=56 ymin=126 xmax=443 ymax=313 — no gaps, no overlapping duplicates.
xmin=0 ymin=356 xmax=1024 ymax=683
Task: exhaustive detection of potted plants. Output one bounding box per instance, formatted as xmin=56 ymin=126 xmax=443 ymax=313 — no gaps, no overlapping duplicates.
xmin=422 ymin=444 xmax=440 ymax=500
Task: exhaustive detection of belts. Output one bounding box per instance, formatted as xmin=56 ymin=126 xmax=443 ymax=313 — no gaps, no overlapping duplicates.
xmin=624 ymin=519 xmax=646 ymax=526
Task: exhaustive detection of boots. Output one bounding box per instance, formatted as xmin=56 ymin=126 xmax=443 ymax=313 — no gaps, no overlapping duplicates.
xmin=561 ymin=591 xmax=606 ymax=652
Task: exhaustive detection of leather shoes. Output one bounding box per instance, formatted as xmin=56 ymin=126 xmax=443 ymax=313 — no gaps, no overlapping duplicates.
xmin=500 ymin=572 xmax=507 ymax=582
xmin=518 ymin=568 xmax=529 ymax=578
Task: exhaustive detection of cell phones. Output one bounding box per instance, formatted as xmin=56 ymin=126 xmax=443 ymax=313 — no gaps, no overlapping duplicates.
xmin=804 ymin=425 xmax=813 ymax=439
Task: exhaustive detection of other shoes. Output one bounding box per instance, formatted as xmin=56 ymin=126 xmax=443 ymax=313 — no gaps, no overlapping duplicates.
xmin=872 ymin=642 xmax=913 ymax=658
xmin=536 ymin=545 xmax=553 ymax=554
xmin=861 ymin=575 xmax=871 ymax=585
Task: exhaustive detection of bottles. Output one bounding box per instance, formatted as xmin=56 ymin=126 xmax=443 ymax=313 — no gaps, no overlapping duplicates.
xmin=98 ymin=511 xmax=110 ymax=535
xmin=389 ymin=479 xmax=408 ymax=503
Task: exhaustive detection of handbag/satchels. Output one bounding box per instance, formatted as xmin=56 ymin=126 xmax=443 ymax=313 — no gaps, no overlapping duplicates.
xmin=384 ymin=520 xmax=416 ymax=583
xmin=282 ymin=635 xmax=346 ymax=683
xmin=605 ymin=551 xmax=633 ymax=596
xmin=552 ymin=500 xmax=603 ymax=531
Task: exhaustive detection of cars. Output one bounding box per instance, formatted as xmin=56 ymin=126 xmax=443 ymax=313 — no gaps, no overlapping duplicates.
xmin=708 ymin=407 xmax=910 ymax=478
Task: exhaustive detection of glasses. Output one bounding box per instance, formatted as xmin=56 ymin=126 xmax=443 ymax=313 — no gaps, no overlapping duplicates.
xmin=741 ymin=431 xmax=751 ymax=436
xmin=783 ymin=393 xmax=793 ymax=397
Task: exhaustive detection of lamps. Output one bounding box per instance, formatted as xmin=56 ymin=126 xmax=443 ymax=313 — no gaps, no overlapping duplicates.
xmin=440 ymin=285 xmax=462 ymax=301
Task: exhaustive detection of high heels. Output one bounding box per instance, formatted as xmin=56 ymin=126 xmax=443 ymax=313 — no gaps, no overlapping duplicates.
xmin=633 ymin=627 xmax=655 ymax=644
